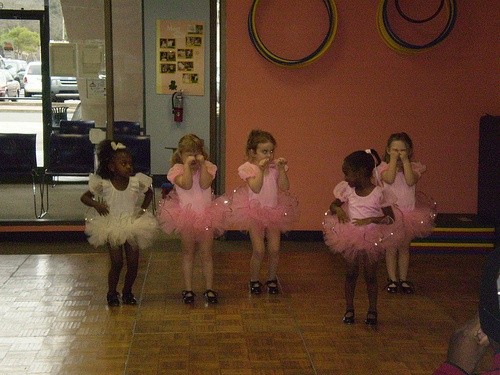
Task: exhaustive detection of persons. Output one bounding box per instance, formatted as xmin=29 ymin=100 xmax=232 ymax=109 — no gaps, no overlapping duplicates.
xmin=377 ymin=132 xmax=437 ymax=295
xmin=322 ymin=148 xmax=395 ymax=326
xmin=156 ymin=134 xmax=229 ymax=304
xmin=431 ymin=248 xmax=500 ymax=375
xmin=80 ymin=140 xmax=159 ymax=306
xmin=231 ymin=130 xmax=299 ymax=295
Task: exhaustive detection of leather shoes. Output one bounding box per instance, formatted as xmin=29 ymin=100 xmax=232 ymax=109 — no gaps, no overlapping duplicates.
xmin=266 ymin=280 xmax=279 ymax=294
xmin=399 ymin=281 xmax=413 ymax=294
xmin=183 ymin=291 xmax=194 ymax=304
xmin=364 ymin=311 xmax=378 ymax=325
xmin=387 ymin=282 xmax=398 ymax=294
xmin=343 ymin=310 xmax=354 ymax=323
xmin=204 ymin=290 xmax=217 ymax=304
xmin=250 ymin=281 xmax=261 ymax=294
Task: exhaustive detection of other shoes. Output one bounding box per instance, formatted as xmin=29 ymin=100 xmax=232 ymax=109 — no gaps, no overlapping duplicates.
xmin=106 ymin=292 xmax=121 ymax=306
xmin=122 ymin=288 xmax=136 ymax=304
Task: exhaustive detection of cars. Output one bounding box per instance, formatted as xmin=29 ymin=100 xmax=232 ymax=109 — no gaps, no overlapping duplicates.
xmin=0 ymin=55 xmax=28 ymax=102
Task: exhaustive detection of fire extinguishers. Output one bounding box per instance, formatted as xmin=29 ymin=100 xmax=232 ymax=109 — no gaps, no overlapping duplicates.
xmin=171 ymin=89 xmax=185 ymax=123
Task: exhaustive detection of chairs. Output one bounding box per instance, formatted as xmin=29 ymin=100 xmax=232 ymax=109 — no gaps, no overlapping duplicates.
xmin=0 ymin=121 xmax=155 ymax=219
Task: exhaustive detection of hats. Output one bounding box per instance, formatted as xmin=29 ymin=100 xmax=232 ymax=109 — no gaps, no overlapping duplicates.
xmin=479 ymin=247 xmax=500 ymax=342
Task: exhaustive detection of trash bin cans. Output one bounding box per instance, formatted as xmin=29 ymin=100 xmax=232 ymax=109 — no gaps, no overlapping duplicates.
xmin=51 ymin=106 xmax=70 ymax=128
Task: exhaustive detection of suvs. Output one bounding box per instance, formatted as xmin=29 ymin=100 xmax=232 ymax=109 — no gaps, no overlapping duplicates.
xmin=50 ymin=75 xmax=80 ymax=103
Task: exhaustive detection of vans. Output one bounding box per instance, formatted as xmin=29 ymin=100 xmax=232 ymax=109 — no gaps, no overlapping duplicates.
xmin=22 ymin=62 xmax=42 ymax=97
